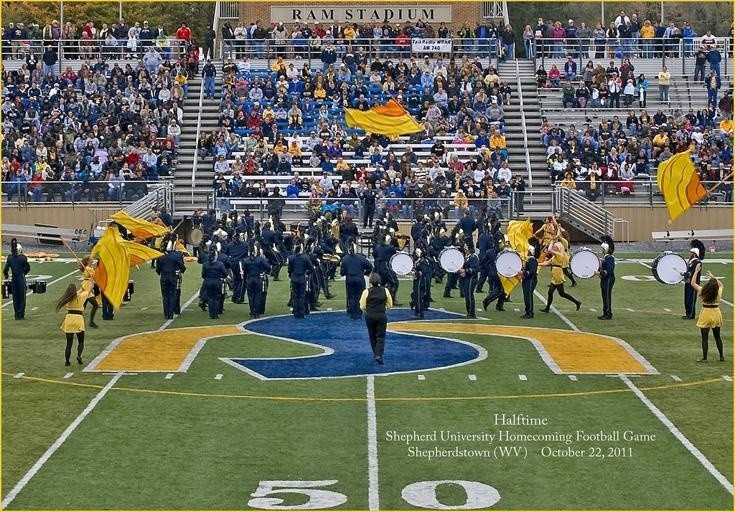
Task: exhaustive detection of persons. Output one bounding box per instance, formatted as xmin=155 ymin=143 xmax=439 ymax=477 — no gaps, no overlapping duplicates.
xmin=359 ymin=272 xmax=393 ymax=365
xmin=58 ymin=284 xmax=98 ymax=367
xmin=690 ymin=262 xmax=726 ymax=362
xmin=1 ymin=10 xmax=732 ymax=330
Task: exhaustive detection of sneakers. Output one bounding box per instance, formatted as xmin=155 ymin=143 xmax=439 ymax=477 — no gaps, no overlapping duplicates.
xmin=539 ymin=308 xmax=549 ymax=313
xmin=64 ymin=356 xmax=82 ymax=366
xmin=696 ymin=354 xmax=724 ymax=362
xmin=198 ymin=295 xmax=225 ymax=319
xmin=434 ymin=279 xmax=484 ymax=298
xmin=466 ymin=299 xmax=512 ymax=319
xmin=89 ymin=321 xmax=98 ymax=328
xmin=270 ymin=272 xmax=282 ymax=281
xmin=572 ymin=283 xmax=577 ymax=286
xmin=375 ymin=355 xmax=384 ymax=364
xmin=682 ymin=315 xmax=691 ymax=319
xmin=294 ymin=284 xmax=335 ymax=319
xmin=409 ymin=298 xmax=437 ymax=319
xmin=248 ymin=312 xmax=261 ymax=318
xmin=597 ymin=316 xmax=611 ymax=320
xmin=231 ymin=299 xmax=248 ymax=304
xmin=576 ymin=302 xmax=580 ymax=310
xmin=392 ymin=300 xmax=403 ymax=305
xmin=520 ymin=314 xmax=534 ymax=319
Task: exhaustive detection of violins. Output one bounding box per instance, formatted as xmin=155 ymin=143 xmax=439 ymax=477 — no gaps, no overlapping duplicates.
xmin=322 ymin=253 xmax=341 ymax=263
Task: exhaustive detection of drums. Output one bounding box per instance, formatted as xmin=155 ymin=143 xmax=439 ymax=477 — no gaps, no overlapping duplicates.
xmin=438 ymin=248 xmax=465 ymax=273
xmin=652 ymin=250 xmax=688 ymax=285
xmin=388 ymin=253 xmax=413 ymax=275
xmin=495 ymin=249 xmax=523 ymax=278
xmin=569 ymin=247 xmax=601 ymax=279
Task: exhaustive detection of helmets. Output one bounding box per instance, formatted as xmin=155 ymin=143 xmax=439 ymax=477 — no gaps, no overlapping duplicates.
xmin=451 ymin=228 xmax=471 ymax=250
xmin=483 ymin=222 xmax=509 ymax=251
xmin=161 ymin=239 xmax=177 ymax=252
xmin=294 ymin=215 xmax=331 ymax=255
xmin=690 ymin=247 xmax=700 ymax=258
xmin=348 ymin=242 xmax=357 ymax=254
xmin=203 ymin=215 xmax=263 ymax=263
xmin=601 ymin=242 xmax=610 ymax=254
xmin=377 ymin=212 xmax=396 ymax=245
xmin=527 ymin=244 xmax=536 ymax=256
xmin=412 ymin=212 xmax=445 ymax=263
xmin=10 ymin=243 xmax=24 ymax=256
